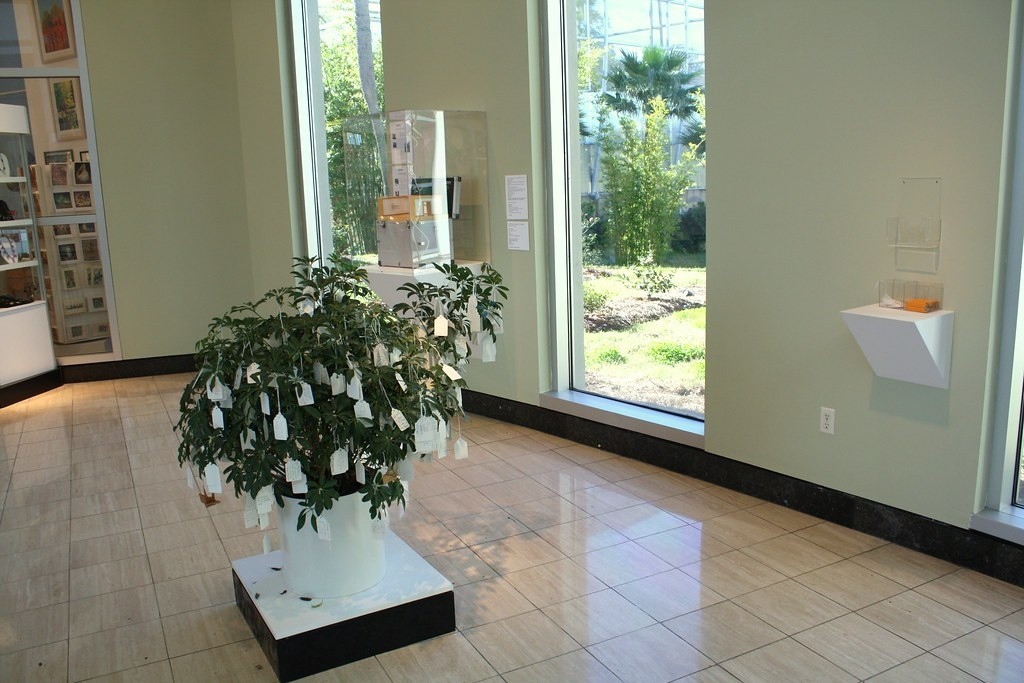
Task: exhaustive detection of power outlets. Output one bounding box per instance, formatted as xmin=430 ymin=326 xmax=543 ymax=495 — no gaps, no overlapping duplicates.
xmin=820 ymin=407 xmax=835 ymax=436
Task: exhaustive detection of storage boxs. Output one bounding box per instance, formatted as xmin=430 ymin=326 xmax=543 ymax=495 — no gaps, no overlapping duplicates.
xmin=904 ymin=281 xmax=944 ymax=314
xmin=879 ymin=279 xmax=918 ymax=308
xmin=341 ymin=107 xmax=491 ymax=277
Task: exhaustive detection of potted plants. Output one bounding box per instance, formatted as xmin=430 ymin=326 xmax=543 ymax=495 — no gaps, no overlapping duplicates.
xmin=172 ymin=253 xmax=510 ymax=598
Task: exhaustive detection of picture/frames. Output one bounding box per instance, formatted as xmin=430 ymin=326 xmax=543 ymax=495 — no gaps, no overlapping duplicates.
xmin=18 ymin=148 xmax=111 ymax=344
xmin=47 ymin=78 xmax=86 ymax=142
xmin=33 ymin=0 xmax=77 ymax=65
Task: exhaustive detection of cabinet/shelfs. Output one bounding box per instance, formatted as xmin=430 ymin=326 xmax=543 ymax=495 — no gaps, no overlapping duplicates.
xmin=16 ymin=162 xmax=115 ymax=356
xmin=0 ymin=176 xmax=45 ymax=313
